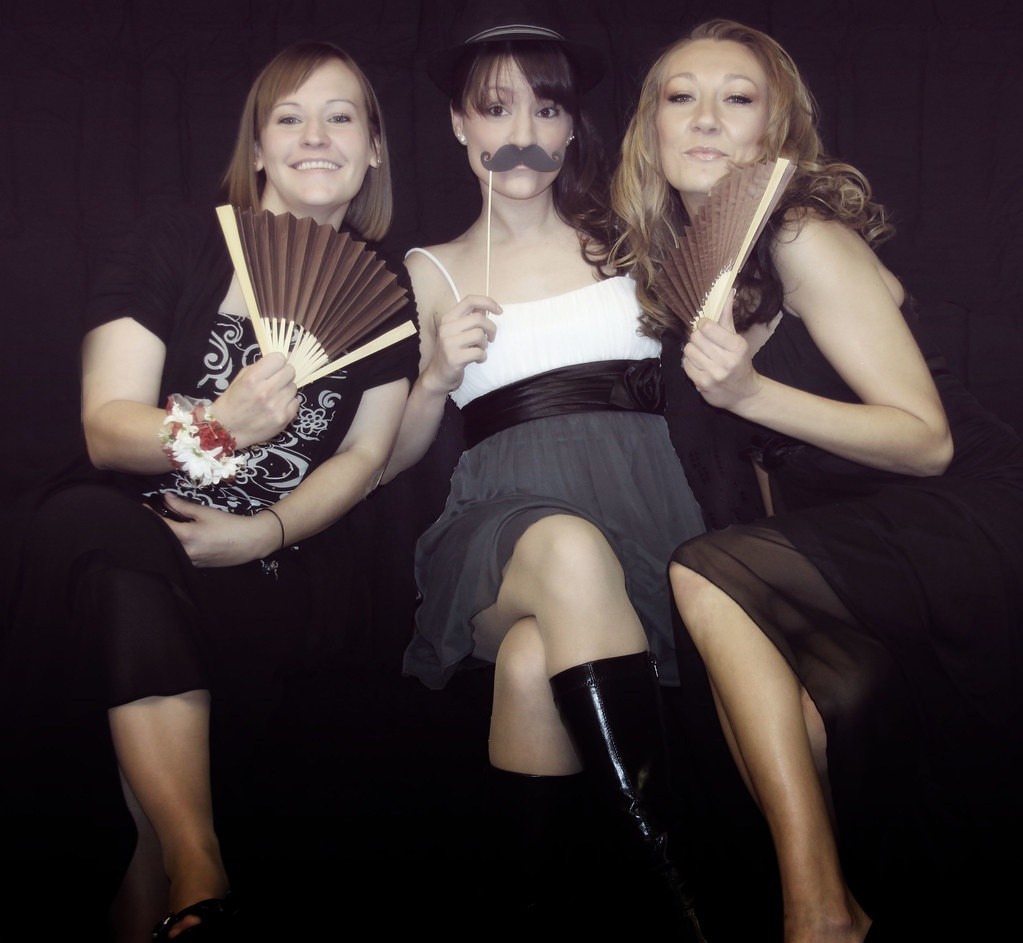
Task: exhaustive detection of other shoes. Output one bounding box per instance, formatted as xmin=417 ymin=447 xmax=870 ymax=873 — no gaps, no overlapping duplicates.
xmin=152 ymin=893 xmax=249 ymax=943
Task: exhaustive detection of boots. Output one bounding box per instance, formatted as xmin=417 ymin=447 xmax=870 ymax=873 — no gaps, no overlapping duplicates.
xmin=550 ymin=648 xmax=724 ymax=943
xmin=486 ymin=762 xmax=580 ymax=943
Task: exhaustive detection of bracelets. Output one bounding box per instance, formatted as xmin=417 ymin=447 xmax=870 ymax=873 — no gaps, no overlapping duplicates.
xmin=260 ymin=509 xmax=285 ymax=553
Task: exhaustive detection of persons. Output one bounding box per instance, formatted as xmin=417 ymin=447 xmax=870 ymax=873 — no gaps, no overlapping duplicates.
xmin=603 ymin=20 xmax=1023 ymax=943
xmin=399 ymin=15 xmax=737 ymax=943
xmin=60 ymin=33 xmax=427 ymax=943
